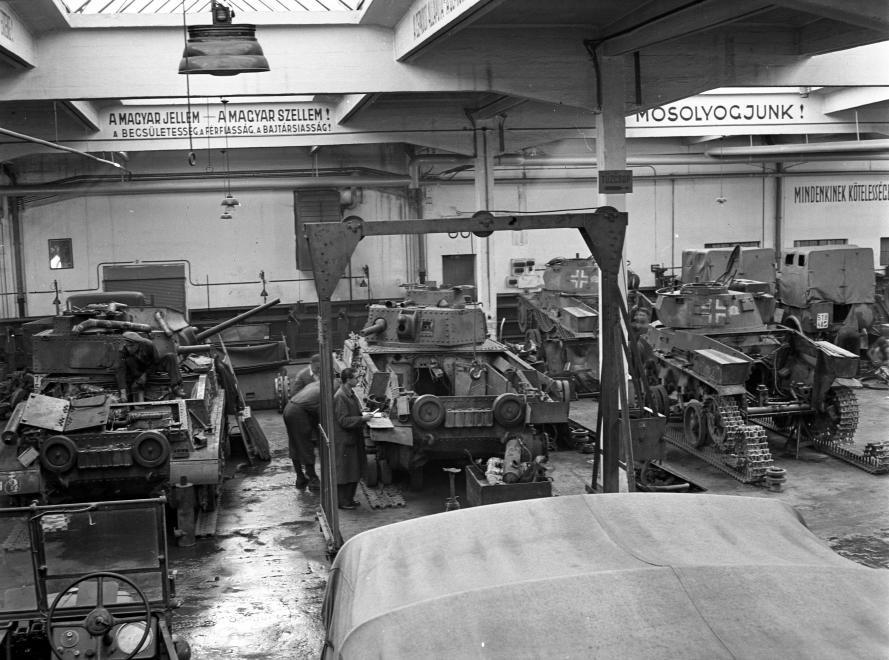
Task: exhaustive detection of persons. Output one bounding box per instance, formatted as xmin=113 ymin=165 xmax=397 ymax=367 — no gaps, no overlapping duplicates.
xmin=115 ymin=331 xmax=187 ymax=410
xmin=332 ymin=367 xmax=375 ymax=511
xmin=282 ymin=374 xmax=342 ymax=492
xmin=287 ymin=355 xmax=324 ymax=398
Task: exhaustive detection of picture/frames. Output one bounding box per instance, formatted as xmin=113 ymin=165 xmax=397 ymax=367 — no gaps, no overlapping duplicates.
xmin=47 ymin=238 xmax=73 ymax=270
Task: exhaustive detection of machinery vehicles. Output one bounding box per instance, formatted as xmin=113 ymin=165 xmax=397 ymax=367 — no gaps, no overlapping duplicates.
xmin=333 ymin=281 xmax=572 ymax=486
xmin=514 ymin=254 xmax=657 ymax=397
xmin=637 ymin=245 xmax=889 ymax=485
xmin=2 ymin=300 xmax=282 ymax=508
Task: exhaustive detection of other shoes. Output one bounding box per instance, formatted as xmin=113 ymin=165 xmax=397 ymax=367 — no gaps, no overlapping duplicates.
xmin=308 ymin=481 xmax=321 ymax=490
xmin=339 ymin=505 xmax=356 ymax=509
xmin=352 ymin=501 xmax=360 ymax=505
xmin=296 ymin=478 xmax=308 ymax=488
